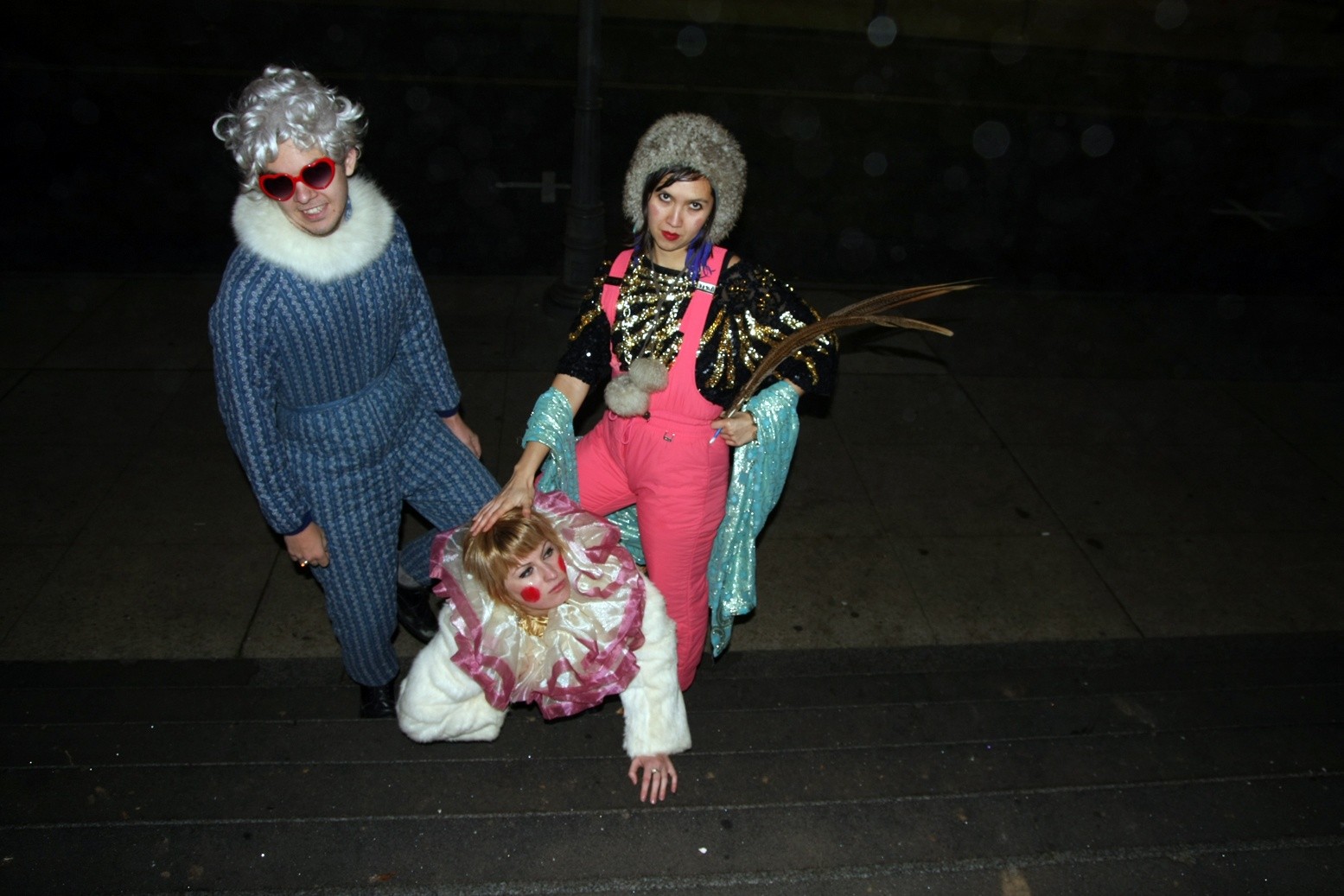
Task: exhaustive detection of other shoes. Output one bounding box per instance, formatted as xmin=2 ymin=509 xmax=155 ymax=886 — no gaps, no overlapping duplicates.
xmin=392 ymin=588 xmax=443 ymax=646
xmin=359 ymin=682 xmax=399 ymax=720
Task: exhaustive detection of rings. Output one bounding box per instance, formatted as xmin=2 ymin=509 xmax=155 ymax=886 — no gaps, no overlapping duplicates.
xmin=651 ymin=769 xmax=660 ymax=773
xmin=298 ymin=559 xmax=308 ymax=568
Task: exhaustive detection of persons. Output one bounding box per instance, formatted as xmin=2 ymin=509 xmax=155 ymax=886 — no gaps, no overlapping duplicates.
xmin=395 ymin=490 xmax=692 ymax=803
xmin=210 ymin=64 xmax=504 ymax=718
xmin=470 ymin=108 xmax=839 ymax=694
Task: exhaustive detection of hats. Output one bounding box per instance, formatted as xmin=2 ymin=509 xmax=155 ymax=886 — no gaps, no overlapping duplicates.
xmin=620 ymin=107 xmax=746 ymax=247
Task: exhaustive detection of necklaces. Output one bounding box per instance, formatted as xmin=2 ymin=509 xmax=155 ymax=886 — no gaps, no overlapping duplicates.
xmin=636 ymin=243 xmax=696 ymax=360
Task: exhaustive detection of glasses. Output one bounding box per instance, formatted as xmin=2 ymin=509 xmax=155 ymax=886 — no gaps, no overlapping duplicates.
xmin=256 ymin=151 xmax=349 ymax=203
